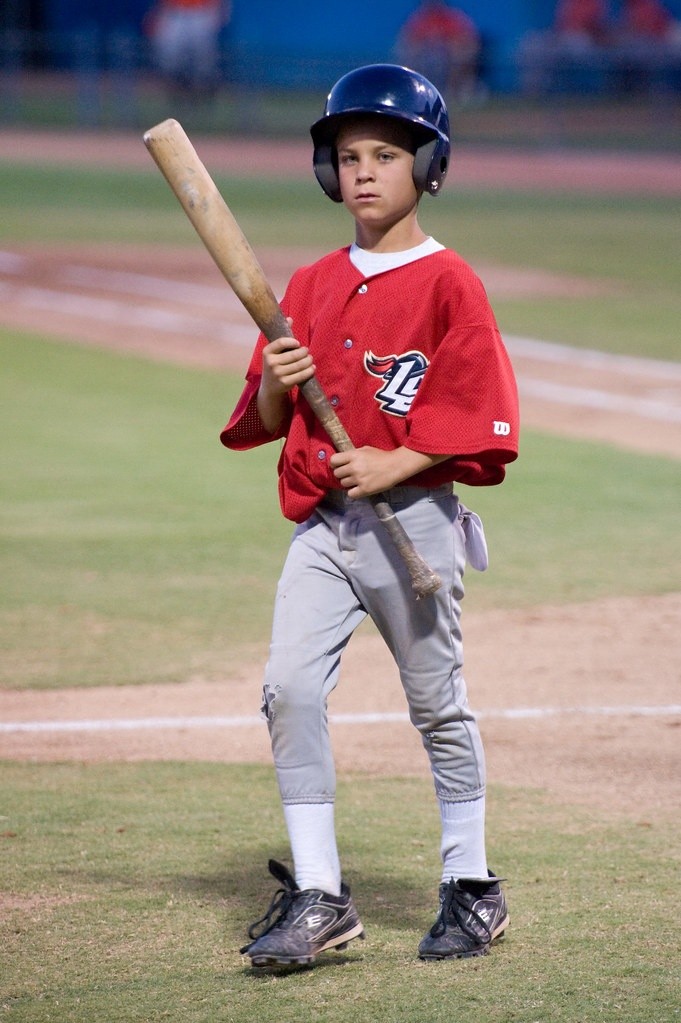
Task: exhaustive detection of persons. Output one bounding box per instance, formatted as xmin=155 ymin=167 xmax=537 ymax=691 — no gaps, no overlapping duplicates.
xmin=146 ymin=0 xmax=681 ymax=104
xmin=220 ymin=63 xmax=518 ymax=968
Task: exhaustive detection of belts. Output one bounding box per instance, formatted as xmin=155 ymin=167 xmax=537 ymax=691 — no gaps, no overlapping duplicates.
xmin=331 ymin=479 xmax=456 ymax=506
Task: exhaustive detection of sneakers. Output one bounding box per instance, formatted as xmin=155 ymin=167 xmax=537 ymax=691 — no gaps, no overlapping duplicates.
xmin=239 ymin=859 xmax=367 ymax=966
xmin=417 ymin=870 xmax=510 ymax=961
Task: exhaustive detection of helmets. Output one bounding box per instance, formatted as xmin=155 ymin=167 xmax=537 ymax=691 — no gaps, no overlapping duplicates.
xmin=310 ymin=62 xmax=452 ymax=203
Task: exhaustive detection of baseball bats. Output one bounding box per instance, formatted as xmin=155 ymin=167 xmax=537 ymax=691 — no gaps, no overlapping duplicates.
xmin=143 ymin=118 xmax=444 ymax=600
